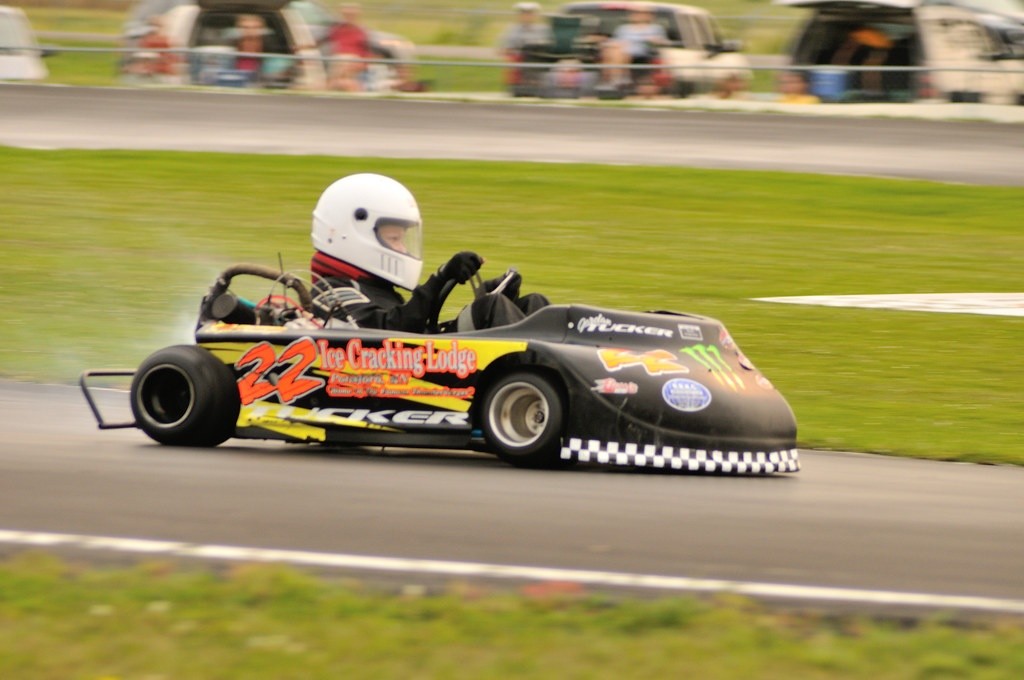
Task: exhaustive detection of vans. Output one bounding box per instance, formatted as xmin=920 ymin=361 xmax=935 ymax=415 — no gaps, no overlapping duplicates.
xmin=777 ymin=0 xmax=1024 ymax=107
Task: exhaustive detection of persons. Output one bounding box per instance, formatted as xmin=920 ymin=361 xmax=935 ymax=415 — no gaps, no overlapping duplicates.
xmin=124 ymin=16 xmax=182 ymax=85
xmin=592 ymin=10 xmax=667 ymax=98
xmin=314 ymin=5 xmax=368 ymax=91
xmin=497 ymin=4 xmax=584 ymax=97
xmin=234 ymin=15 xmax=318 ymax=91
xmin=309 ymin=173 xmax=550 ymax=338
xmin=686 ymin=51 xmax=822 ymax=104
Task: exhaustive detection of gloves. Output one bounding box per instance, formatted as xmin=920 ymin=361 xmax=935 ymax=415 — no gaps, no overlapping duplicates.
xmin=480 ymin=268 xmax=521 ymax=300
xmin=438 ymin=252 xmax=484 ymax=286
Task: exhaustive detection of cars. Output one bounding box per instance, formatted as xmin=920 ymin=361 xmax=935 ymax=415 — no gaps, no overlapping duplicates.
xmin=113 ymin=0 xmax=421 ymax=93
xmin=0 ymin=7 xmax=67 ymax=83
xmin=514 ymin=3 xmax=753 ymax=100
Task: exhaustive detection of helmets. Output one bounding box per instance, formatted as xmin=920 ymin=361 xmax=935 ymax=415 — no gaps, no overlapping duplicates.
xmin=310 ymin=173 xmax=423 ymax=291
